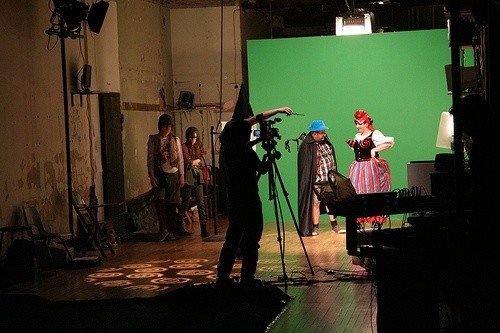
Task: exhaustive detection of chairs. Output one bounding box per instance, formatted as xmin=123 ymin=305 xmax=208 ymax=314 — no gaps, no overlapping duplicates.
xmin=72 ymin=191 xmax=115 ymax=257
xmin=23 ymin=206 xmax=74 ymax=272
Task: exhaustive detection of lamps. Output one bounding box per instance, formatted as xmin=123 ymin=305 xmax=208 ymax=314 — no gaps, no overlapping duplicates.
xmin=53 ymin=0 xmax=110 ymax=35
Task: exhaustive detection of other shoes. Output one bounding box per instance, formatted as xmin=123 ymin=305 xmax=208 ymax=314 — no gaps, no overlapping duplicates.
xmin=200 ymin=224 xmax=211 ymax=238
xmin=242 ymin=279 xmax=262 ymax=287
xmin=311 ymin=227 xmax=319 ymax=236
xmin=331 ymin=225 xmax=346 ymax=234
xmin=161 ymin=233 xmax=176 ymax=241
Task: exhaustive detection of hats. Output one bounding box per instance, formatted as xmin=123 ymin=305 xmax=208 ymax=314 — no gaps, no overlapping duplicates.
xmin=189 ymin=132 xmax=198 ymax=138
xmin=307 ymin=120 xmax=328 ymax=131
xmin=158 ymin=113 xmax=174 ymax=131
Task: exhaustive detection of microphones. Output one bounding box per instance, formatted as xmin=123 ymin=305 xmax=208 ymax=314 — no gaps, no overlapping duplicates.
xmin=275 ymin=118 xmax=282 ymax=122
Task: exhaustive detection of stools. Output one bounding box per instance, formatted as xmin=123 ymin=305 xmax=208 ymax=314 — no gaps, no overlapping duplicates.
xmin=0 ymin=224 xmax=42 ymax=282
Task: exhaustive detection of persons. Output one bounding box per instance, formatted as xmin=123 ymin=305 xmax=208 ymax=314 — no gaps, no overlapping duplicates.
xmin=215 ymin=107 xmax=393 ymax=283
xmin=147 ymin=114 xmax=212 ymax=240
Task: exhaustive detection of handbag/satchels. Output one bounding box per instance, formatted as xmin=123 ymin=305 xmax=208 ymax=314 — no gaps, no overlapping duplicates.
xmin=312 ymin=170 xmax=356 ymax=205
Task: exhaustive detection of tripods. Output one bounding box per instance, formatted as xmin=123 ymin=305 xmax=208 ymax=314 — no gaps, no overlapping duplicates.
xmin=257 ymin=154 xmax=315 ymax=290
